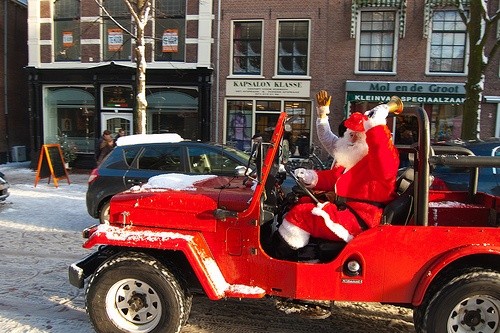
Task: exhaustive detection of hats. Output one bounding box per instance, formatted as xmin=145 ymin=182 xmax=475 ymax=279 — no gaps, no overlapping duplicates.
xmin=251 ymin=134 xmax=262 ymax=139
xmin=344 ymin=112 xmax=365 ymax=132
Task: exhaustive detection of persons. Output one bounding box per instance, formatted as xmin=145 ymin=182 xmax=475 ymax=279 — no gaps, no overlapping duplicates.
xmin=96 ymin=139 xmax=114 ymax=166
xmin=251 ymin=134 xmax=268 ymax=158
xmin=230 ymin=111 xmax=252 ymax=140
xmin=114 ymin=129 xmax=125 ymax=143
xmin=98 ymin=130 xmax=112 ymax=150
xmin=275 ymin=90 xmax=348 ymax=228
xmin=270 ymin=104 xmax=400 ymax=263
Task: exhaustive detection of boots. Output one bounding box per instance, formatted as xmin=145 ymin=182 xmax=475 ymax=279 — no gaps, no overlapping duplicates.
xmin=263 ymin=230 xmax=296 ymax=260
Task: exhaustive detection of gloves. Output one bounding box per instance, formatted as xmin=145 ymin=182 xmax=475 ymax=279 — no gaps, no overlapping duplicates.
xmin=368 ymin=104 xmax=390 ymax=120
xmin=294 ymin=168 xmax=313 ymax=184
xmin=315 ymin=90 xmax=333 ymax=114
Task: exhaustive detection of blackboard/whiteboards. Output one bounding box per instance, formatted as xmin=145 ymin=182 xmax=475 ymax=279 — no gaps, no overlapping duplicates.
xmin=33 ymin=142 xmax=71 ymax=189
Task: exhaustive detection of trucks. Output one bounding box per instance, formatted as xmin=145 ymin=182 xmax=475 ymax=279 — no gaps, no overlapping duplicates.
xmin=67 ymin=103 xmax=500 ymax=333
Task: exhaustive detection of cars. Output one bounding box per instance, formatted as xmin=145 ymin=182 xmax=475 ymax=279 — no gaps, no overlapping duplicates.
xmin=429 ymin=138 xmax=500 ymax=196
xmin=85 ymin=138 xmax=299 ymax=225
